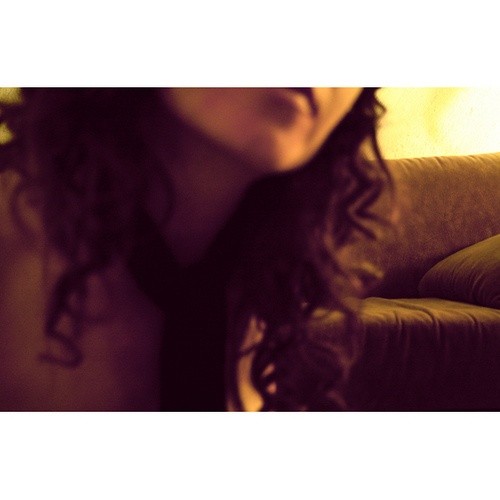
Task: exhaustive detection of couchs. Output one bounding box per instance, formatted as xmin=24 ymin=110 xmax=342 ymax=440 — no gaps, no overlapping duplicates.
xmin=306 ymin=151 xmax=499 ymax=412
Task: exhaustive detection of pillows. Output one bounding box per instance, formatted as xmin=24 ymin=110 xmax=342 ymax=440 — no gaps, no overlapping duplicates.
xmin=418 ymin=233 xmax=500 ymax=310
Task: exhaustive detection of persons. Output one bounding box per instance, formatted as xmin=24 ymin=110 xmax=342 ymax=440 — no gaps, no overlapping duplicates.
xmin=0 ymin=88 xmax=403 ymax=413
